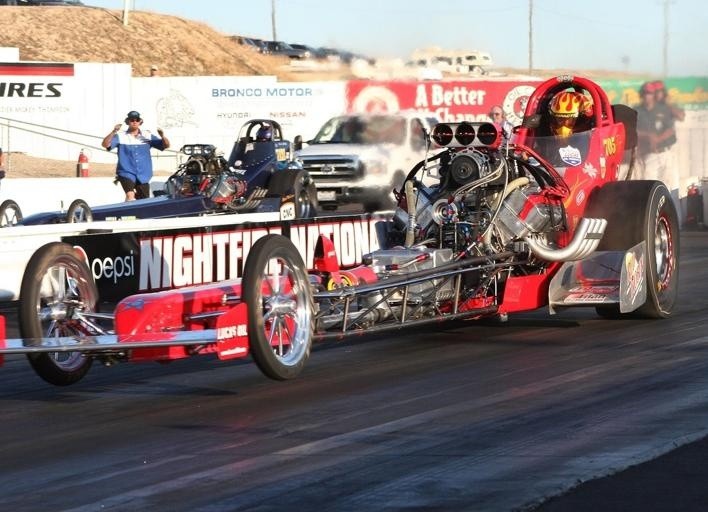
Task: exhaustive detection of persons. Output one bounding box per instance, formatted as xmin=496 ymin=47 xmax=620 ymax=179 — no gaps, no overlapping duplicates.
xmin=255 ymin=126 xmax=274 ymax=142
xmin=101 ymin=111 xmax=170 ymax=201
xmin=548 ymin=92 xmax=591 ymax=159
xmin=489 ymin=106 xmax=515 ymax=138
xmin=651 ymin=79 xmax=686 ymax=191
xmin=632 ymin=82 xmax=674 ymax=185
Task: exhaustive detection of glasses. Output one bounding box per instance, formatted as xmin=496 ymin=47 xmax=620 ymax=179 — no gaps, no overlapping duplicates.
xmin=130 ymin=119 xmax=140 ymax=122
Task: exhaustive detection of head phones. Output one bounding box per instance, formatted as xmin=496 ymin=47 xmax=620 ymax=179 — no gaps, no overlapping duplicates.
xmin=488 ymin=105 xmax=505 ymax=118
xmin=125 ymin=110 xmax=142 ymax=122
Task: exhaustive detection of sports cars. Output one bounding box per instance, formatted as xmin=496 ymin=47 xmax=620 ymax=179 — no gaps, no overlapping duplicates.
xmin=0 ymin=69 xmax=683 ymax=387
xmin=0 ymin=114 xmax=325 ymax=227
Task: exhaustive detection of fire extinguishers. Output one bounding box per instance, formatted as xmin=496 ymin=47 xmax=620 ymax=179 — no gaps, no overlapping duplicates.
xmin=79 ymin=148 xmax=88 ymax=177
xmin=686 ymin=183 xmax=703 ymax=226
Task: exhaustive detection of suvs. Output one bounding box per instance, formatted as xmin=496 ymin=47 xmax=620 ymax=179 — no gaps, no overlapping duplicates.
xmin=226 ymin=35 xmax=378 ymax=68
xmin=290 ymin=104 xmax=449 ymax=214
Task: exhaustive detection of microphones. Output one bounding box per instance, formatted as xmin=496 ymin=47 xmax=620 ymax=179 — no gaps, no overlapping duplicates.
xmin=140 ymin=118 xmax=143 ymax=125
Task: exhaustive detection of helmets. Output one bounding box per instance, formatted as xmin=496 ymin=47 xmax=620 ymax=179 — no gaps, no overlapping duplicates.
xmin=256 ymin=127 xmax=272 ymax=140
xmin=550 ymin=93 xmax=593 ymax=146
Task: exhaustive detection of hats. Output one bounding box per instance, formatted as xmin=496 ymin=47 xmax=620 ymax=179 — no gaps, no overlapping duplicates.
xmin=125 ymin=112 xmax=143 ymax=125
xmin=642 ymin=81 xmax=664 ymax=94
